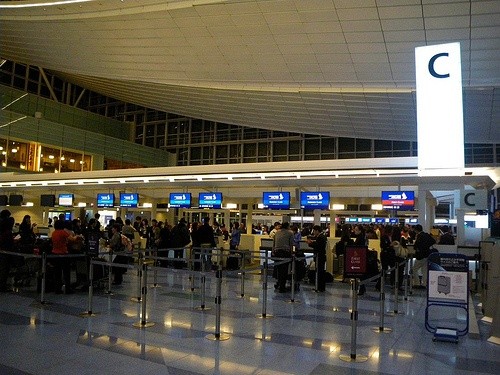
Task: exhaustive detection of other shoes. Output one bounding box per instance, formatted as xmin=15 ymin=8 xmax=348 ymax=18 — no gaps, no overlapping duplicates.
xmin=273 ymin=282 xmax=289 ymax=294
xmin=311 ymin=287 xmax=325 ymax=294
xmin=358 ymin=288 xmax=366 ymax=296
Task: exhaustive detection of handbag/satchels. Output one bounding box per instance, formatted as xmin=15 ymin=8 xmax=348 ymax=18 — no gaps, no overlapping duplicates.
xmin=393 ymin=242 xmax=408 ymax=259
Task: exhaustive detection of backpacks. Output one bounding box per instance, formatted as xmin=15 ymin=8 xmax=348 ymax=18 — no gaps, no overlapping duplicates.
xmin=111 ymin=232 xmax=126 ymax=252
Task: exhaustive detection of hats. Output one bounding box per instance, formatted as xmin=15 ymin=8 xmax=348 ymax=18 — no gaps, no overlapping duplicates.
xmin=179 ymin=218 xmax=188 ymax=223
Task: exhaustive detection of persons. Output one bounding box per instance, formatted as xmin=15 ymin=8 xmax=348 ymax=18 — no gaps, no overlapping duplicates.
xmin=0 ymin=209 xmax=500 ymax=293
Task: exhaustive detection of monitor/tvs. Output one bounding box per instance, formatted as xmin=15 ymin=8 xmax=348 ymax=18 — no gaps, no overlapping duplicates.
xmin=300 ymin=191 xmax=331 ymax=211
xmin=97 ymin=192 xmax=114 ymax=207
xmin=59 ymin=194 xmax=74 ymax=206
xmin=262 ymin=192 xmax=290 ymax=210
xmin=65 ymin=211 xmax=71 ymax=221
xmin=381 ymin=191 xmax=415 ymax=211
xmin=9 ymin=194 xmax=23 ymax=206
xmin=0 ymin=195 xmax=8 ymax=206
xmin=40 ymin=194 xmax=55 ymax=207
xmin=198 ymin=192 xmax=223 ymax=208
xmin=120 ymin=192 xmax=139 ymax=207
xmin=168 ymin=192 xmax=191 ymax=208
